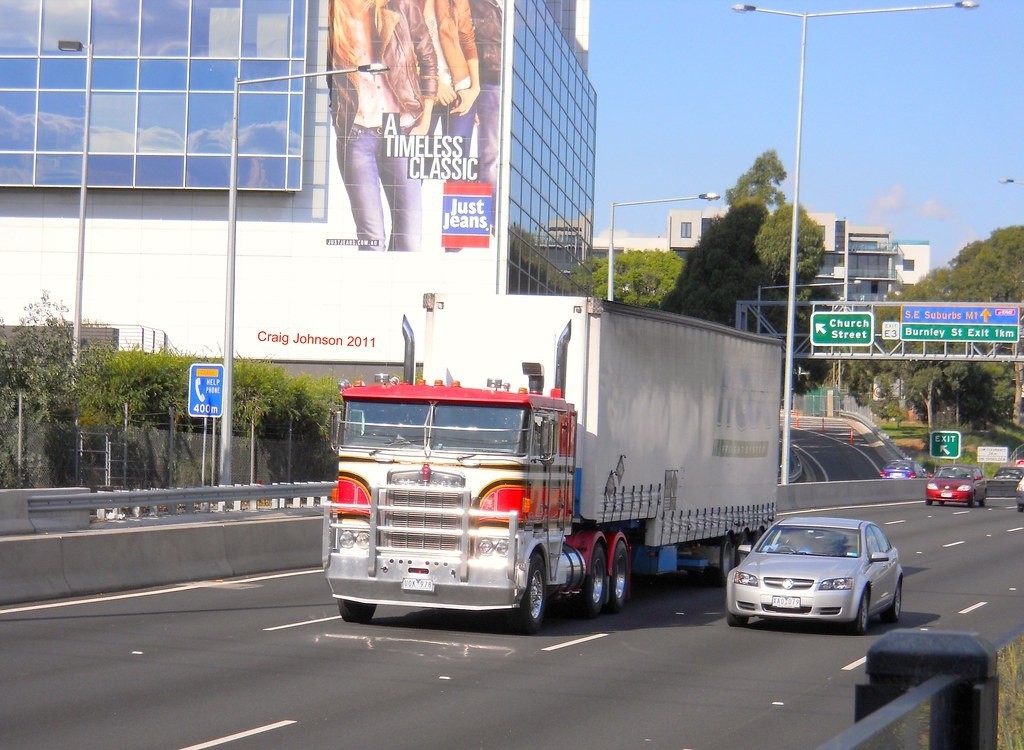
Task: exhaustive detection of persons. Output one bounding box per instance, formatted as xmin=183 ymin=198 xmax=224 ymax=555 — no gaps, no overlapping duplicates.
xmin=327 ymin=0 xmax=503 ymax=251
xmin=822 ymin=533 xmax=857 ymax=557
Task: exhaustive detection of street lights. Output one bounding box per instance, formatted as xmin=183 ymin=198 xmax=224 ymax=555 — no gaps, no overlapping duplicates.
xmin=731 ymin=1 xmax=980 ymax=484
xmin=219 ymin=62 xmax=390 ymax=488
xmin=607 ymin=193 xmax=719 ymax=300
xmin=59 ymin=39 xmax=93 ymax=366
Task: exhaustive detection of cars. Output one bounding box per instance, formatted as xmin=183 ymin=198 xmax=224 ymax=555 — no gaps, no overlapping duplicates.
xmin=925 ymin=464 xmax=987 ymax=509
xmin=882 ymin=462 xmax=917 ymax=479
xmin=994 ymin=466 xmax=1024 ymax=479
xmin=724 ymin=516 xmax=904 ymax=634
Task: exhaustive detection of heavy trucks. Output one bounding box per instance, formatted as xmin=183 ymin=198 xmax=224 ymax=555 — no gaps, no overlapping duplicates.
xmin=322 ymin=289 xmax=783 ymax=637
